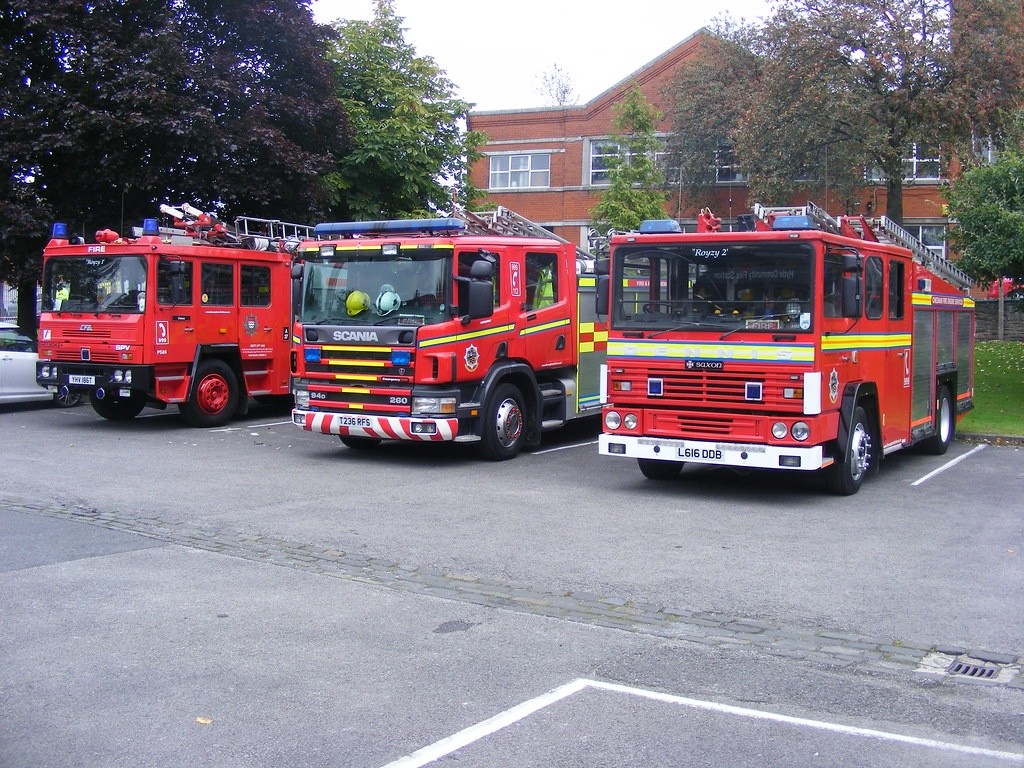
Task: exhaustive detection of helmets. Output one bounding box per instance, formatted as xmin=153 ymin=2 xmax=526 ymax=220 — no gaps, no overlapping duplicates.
xmin=345 ymin=290 xmax=370 ymax=316
xmin=376 ymin=291 xmax=402 ymax=317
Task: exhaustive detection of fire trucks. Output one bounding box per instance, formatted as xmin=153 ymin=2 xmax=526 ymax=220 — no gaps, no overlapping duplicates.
xmin=285 ymin=203 xmax=700 ymax=463
xmin=35 ymin=204 xmax=347 ymax=429
xmin=597 ymin=203 xmax=976 ymax=496
